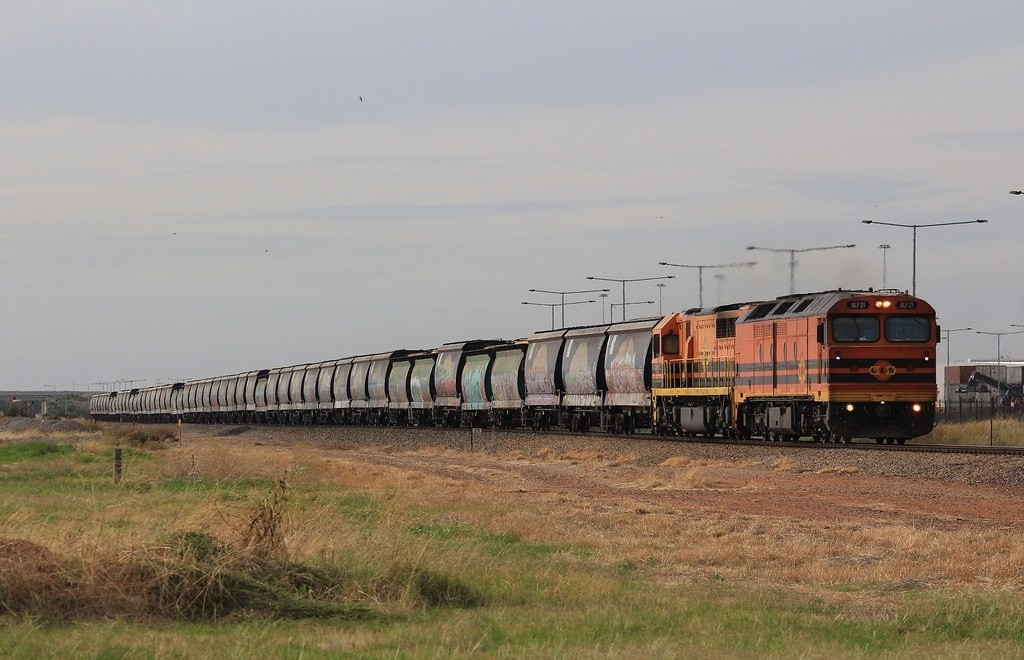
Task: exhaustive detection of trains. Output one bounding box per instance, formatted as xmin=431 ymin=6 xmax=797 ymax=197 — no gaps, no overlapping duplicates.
xmin=89 ymin=284 xmax=938 ymax=444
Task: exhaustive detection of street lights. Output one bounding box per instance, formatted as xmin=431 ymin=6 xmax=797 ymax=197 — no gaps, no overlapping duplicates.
xmin=658 ymin=259 xmax=760 ymax=311
xmin=976 ymin=330 xmax=1023 ymax=408
xmin=582 ymin=275 xmax=676 ymax=322
xmin=44 ymin=378 xmax=193 ymax=390
xmin=1011 ymin=324 xmax=1023 ymax=327
xmin=878 ymin=244 xmax=892 ymax=288
xmin=529 ymin=288 xmax=610 ymax=327
xmin=747 ymin=244 xmax=856 ymax=294
xmin=656 ymin=283 xmax=666 ymax=316
xmin=520 ymin=301 xmax=597 ymax=331
xmin=943 ymin=327 xmax=973 ymax=422
xmin=599 ymin=294 xmax=608 ymax=325
xmin=610 ymin=301 xmax=654 ymax=323
xmin=860 ymin=218 xmax=989 ymax=298
xmin=1009 ymin=190 xmax=1023 ymax=195
xmin=715 ymin=274 xmax=725 ymax=309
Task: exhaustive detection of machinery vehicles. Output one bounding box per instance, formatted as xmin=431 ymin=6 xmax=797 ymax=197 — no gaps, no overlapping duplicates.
xmin=955 ymin=371 xmax=1024 ymax=415
